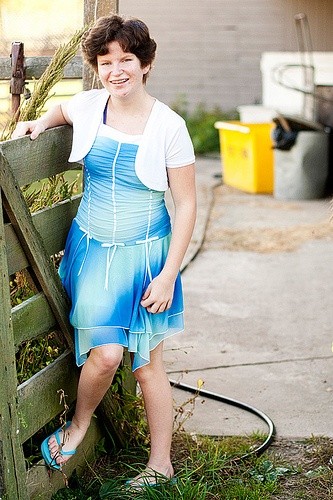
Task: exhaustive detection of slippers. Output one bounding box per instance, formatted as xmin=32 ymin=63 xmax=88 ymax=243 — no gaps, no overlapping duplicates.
xmin=125 ymin=478 xmax=177 ymax=493
xmin=41 ymin=421 xmax=76 ymax=471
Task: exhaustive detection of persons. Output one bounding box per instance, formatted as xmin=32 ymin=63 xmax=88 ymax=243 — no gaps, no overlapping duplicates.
xmin=10 ymin=15 xmax=196 ymax=494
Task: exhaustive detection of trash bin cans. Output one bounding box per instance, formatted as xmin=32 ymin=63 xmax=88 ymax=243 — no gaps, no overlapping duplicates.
xmin=272 ymin=113 xmax=330 ymax=200
